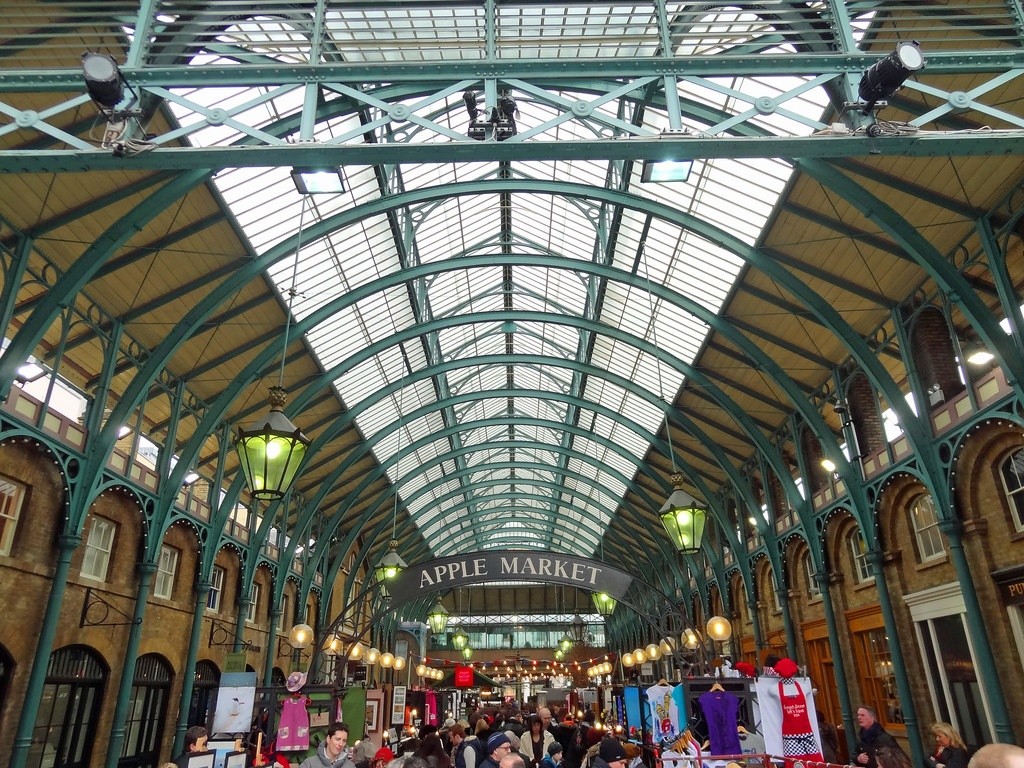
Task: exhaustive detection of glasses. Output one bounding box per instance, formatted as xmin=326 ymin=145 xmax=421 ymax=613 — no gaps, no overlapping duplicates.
xmin=195 ymin=740 xmax=208 ymax=746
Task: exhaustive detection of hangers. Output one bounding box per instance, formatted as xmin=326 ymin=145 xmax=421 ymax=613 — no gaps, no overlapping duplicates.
xmin=709 ymin=676 xmax=725 ymax=692
xmin=656 ymin=676 xmax=672 ymax=687
xmin=280 ymin=691 xmax=311 ymax=705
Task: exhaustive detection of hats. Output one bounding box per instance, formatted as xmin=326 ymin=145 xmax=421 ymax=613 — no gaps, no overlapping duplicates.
xmin=600 ymin=738 xmax=626 ymax=762
xmin=375 ymin=747 xmax=393 ymax=763
xmin=625 ymin=743 xmax=640 ymax=759
xmin=286 ymin=672 xmax=308 ymax=692
xmin=446 ymin=719 xmax=456 ymax=727
xmin=547 ymin=741 xmax=563 ymax=755
xmin=737 ymin=662 xmax=755 ymax=676
xmin=487 ymin=732 xmax=511 ymax=753
xmin=419 ymin=724 xmax=437 ymax=740
xmin=587 ymin=728 xmax=606 ymax=744
xmin=774 ymin=658 xmax=798 ymax=678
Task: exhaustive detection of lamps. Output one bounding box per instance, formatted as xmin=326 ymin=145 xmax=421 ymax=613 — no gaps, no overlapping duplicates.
xmin=290 ymin=166 xmax=346 ymax=194
xmin=279 ymin=624 xmax=445 ymax=687
xmin=586 ymin=616 xmax=734 ymax=680
xmin=75 ymin=53 xmax=155 ymax=150
xmin=551 ymin=417 xmax=619 ymax=658
xmin=375 ymin=340 xmax=476 ymax=659
xmin=610 ymin=161 xmax=711 ymax=555
xmin=234 ymin=188 xmax=311 ymax=500
xmin=858 ymin=38 xmax=926 ymax=136
xmin=640 ymin=159 xmax=694 ymax=183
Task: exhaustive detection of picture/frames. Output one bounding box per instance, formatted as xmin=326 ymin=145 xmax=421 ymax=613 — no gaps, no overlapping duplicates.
xmin=365 ymin=698 xmax=380 ymax=733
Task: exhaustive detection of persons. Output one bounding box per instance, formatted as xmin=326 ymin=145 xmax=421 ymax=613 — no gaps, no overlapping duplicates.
xmin=371 ymin=698 xmax=647 ymax=768
xmin=968 ymin=743 xmax=1024 ymax=768
xmin=160 ymin=726 xmax=208 ymax=768
xmin=876 ymin=749 xmax=912 ymax=768
xmin=298 ymin=723 xmax=356 ymax=768
xmin=249 ymin=709 xmax=275 ymax=753
xmin=852 ymin=706 xmax=900 ymax=768
xmin=816 ymin=711 xmax=838 ymax=763
xmin=930 ymin=723 xmax=969 ymax=768
